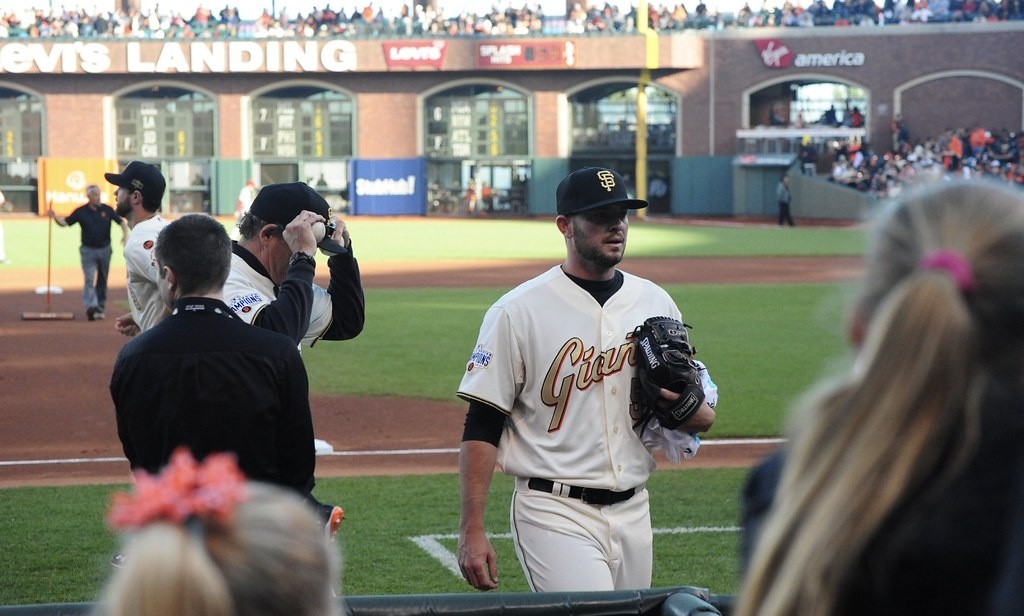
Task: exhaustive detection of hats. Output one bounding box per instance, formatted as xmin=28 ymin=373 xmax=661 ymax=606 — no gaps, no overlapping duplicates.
xmin=105 ymin=161 xmax=167 ymax=200
xmin=557 ymin=166 xmax=648 ymax=215
xmin=250 ymin=181 xmax=333 ymax=230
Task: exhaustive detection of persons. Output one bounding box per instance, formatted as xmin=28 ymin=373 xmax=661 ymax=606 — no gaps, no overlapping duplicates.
xmin=455 ymin=168 xmax=717 ymax=596
xmin=48 ymin=185 xmax=129 ymax=320
xmin=425 ymin=172 xmax=531 ymax=216
xmin=97 ymin=444 xmax=349 ymax=615
xmin=218 ymin=178 xmax=364 ymax=355
xmin=2 ymin=0 xmax=1022 ymax=39
xmin=735 ymin=181 xmax=1023 ymax=616
xmin=777 ymin=177 xmax=795 ymax=225
xmin=109 ymin=214 xmax=317 ymax=511
xmin=762 ymin=98 xmax=1023 ymax=201
xmin=105 ymin=157 xmax=346 ymax=540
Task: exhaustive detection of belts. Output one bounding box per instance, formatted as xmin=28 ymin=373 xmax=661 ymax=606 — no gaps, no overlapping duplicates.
xmin=529 ymin=477 xmax=635 ymax=506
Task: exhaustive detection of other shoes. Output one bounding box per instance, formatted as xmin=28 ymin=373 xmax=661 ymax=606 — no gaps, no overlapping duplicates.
xmin=88 ymin=305 xmax=105 ymax=320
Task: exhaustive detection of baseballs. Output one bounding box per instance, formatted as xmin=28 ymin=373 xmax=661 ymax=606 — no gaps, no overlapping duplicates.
xmin=311 ymin=221 xmax=326 ymax=243
xmin=318 ymin=231 xmax=345 ymax=257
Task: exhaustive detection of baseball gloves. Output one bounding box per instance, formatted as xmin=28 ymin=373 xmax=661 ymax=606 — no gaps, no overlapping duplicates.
xmin=637 ymin=316 xmax=704 ymax=428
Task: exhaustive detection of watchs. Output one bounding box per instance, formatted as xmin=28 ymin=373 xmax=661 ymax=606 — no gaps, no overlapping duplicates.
xmin=288 ymin=251 xmax=316 ymax=269
